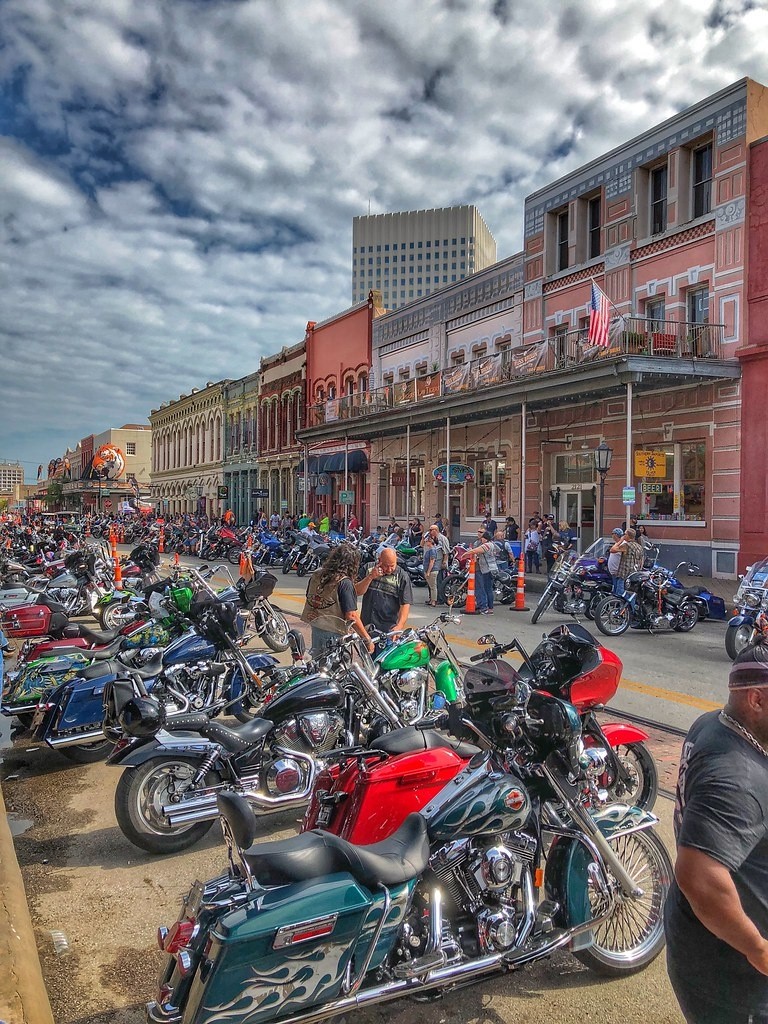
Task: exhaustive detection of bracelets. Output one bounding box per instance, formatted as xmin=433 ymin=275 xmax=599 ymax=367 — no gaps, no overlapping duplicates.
xmin=442 ymin=562 xmax=447 ymax=565
xmin=568 ymin=546 xmax=571 ymax=548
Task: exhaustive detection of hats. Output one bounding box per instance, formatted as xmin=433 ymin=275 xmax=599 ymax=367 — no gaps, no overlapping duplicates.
xmin=485 ymin=513 xmax=491 ymax=517
xmin=435 ymin=513 xmax=441 ymax=518
xmin=729 ymin=636 xmax=768 ymax=691
xmin=612 ymin=527 xmax=624 ymax=537
xmin=308 ymin=522 xmax=316 ymax=527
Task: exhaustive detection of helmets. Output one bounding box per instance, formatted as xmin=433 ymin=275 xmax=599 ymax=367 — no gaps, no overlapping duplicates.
xmin=483 ymin=531 xmax=493 ymax=540
xmin=546 ymin=514 xmax=554 ymax=520
xmin=529 ymin=520 xmax=537 ymax=525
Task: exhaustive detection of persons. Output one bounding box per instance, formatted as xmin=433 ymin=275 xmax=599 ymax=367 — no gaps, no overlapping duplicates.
xmin=433 ymin=514 xmax=443 ymax=529
xmin=610 ymin=528 xmax=644 ymax=614
xmin=422 ymin=537 xmax=438 ymax=607
xmin=472 ymin=527 xmax=501 ymax=609
xmin=385 ymin=517 xmax=399 ymax=535
xmin=395 ymin=533 xmax=403 ymax=548
xmin=623 ymin=514 xmax=638 ymax=534
xmin=300 ymin=546 xmax=375 ymax=654
xmin=635 ymin=525 xmax=649 ymax=545
xmin=651 ymin=485 xmax=673 ymax=514
xmin=528 ymin=511 xmax=542 ymax=524
xmin=373 ymin=526 xmax=381 ymax=542
xmin=543 ymin=514 xmax=558 ymax=572
xmin=439 ymin=518 xmax=449 ymax=538
xmin=411 ymin=517 xmax=424 ymax=543
xmin=480 ymin=513 xmax=497 ymax=536
xmin=664 ymin=643 xmax=768 ymax=1022
xmin=0 ymin=508 xmax=356 ymax=557
xmin=421 ymin=526 xmax=450 ymax=604
xmin=527 ymin=520 xmax=543 ymax=574
xmin=490 ymin=532 xmax=515 ymax=569
xmin=554 ymin=521 xmax=574 ymax=550
xmin=608 ymin=527 xmax=622 ymax=592
xmin=540 ymin=515 xmax=548 ymax=533
xmin=498 ymin=517 xmax=520 ymax=542
xmin=461 ymin=531 xmax=498 ymax=615
xmin=354 ymin=549 xmax=412 ymax=640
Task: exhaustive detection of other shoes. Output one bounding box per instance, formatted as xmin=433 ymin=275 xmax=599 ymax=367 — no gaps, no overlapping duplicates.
xmin=425 ymin=599 xmax=436 ymax=606
xmin=479 ymin=608 xmax=494 ymax=616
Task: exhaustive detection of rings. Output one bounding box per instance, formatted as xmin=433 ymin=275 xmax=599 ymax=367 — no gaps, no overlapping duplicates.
xmin=378 ymin=567 xmax=382 ymax=573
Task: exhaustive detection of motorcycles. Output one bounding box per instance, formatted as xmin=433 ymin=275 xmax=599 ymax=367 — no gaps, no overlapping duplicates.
xmin=0 ymin=497 xmax=768 ymax=1024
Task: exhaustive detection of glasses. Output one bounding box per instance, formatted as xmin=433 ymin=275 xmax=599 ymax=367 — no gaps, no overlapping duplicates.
xmin=477 ymin=531 xmax=483 ymax=533
xmin=429 ymin=529 xmax=435 ymax=531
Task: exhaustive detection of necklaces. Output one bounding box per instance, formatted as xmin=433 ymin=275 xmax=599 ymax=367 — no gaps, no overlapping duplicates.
xmin=718 ymin=707 xmax=768 ymax=755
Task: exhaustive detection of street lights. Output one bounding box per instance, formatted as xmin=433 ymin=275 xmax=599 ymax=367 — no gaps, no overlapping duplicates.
xmin=98 ymin=464 xmax=102 ymax=511
xmin=593 ymin=439 xmax=615 ymax=560
xmin=27 ymin=487 xmax=30 ymax=516
xmin=132 ymin=482 xmax=140 ymax=516
xmin=80 ymin=495 xmax=83 ymax=516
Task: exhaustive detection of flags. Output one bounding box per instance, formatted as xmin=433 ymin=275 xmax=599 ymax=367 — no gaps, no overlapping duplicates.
xmin=588 ymin=282 xmax=611 ymax=347
